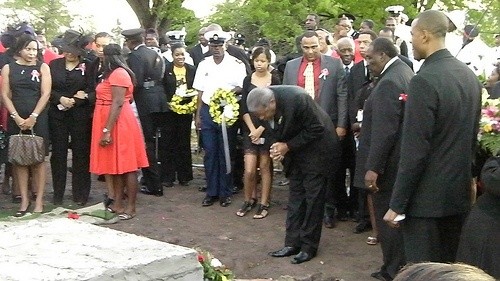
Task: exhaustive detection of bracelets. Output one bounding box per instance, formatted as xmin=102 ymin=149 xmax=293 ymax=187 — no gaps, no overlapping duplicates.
xmin=30 ymin=113 xmax=38 ymax=120
xmin=10 ymin=111 xmax=18 ymax=118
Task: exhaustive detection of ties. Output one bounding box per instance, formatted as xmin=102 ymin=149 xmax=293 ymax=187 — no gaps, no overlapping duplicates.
xmin=345 ymin=67 xmax=349 ymax=76
xmin=365 ymin=65 xmax=370 ymax=79
xmin=303 ymin=61 xmax=315 ymax=100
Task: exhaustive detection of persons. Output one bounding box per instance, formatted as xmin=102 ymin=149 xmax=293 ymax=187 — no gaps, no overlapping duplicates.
xmin=89 ymin=43 xmax=149 ymax=220
xmin=0 ymin=5 xmax=500 ymax=281
xmin=392 ymin=260 xmax=496 ymax=281
xmin=246 ymin=85 xmax=340 ymax=264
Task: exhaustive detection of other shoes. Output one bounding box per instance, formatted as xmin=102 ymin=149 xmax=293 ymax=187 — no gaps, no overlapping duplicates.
xmin=324 ymin=206 xmax=378 ymax=245
xmin=253 ymin=203 xmax=270 ymax=219
xmin=220 ymin=196 xmax=227 ymax=207
xmin=279 ymin=180 xmax=290 ymax=185
xmin=3 ymin=165 xmax=187 ymax=219
xmin=231 ymin=186 xmax=238 ymax=192
xmin=199 ymin=186 xmax=209 ymax=191
xmin=236 ymin=199 xmax=257 ymax=217
xmin=202 ymin=195 xmax=216 ymax=206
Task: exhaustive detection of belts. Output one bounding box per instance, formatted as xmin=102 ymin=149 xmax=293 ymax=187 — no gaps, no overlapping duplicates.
xmin=143 ymin=79 xmax=163 ymax=88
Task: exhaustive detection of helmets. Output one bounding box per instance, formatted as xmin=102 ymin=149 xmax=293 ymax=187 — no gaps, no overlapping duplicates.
xmin=444 ymin=10 xmax=466 ymax=31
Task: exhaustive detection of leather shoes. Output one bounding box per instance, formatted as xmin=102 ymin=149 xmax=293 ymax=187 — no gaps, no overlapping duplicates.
xmin=292 ymin=251 xmax=311 ymax=264
xmin=270 ymin=245 xmax=299 ymax=257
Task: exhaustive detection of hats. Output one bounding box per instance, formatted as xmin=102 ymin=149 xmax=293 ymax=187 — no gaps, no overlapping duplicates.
xmin=235 ymin=34 xmax=245 ymax=40
xmin=52 ymin=30 xmax=88 ymax=56
xmin=121 ymin=30 xmax=143 ymax=41
xmin=385 ymin=6 xmax=404 ymax=17
xmin=399 ymin=11 xmax=409 ymax=22
xmin=165 ymin=30 xmax=187 ymax=42
xmin=204 ymin=30 xmax=232 ymax=47
xmin=337 ymin=12 xmax=354 ymax=23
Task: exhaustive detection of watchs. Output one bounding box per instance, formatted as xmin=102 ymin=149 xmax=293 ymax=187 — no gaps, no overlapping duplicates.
xmin=102 ymin=127 xmax=110 ymax=132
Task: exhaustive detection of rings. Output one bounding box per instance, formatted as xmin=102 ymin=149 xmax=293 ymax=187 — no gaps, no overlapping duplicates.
xmin=368 ymin=184 xmax=373 ymax=188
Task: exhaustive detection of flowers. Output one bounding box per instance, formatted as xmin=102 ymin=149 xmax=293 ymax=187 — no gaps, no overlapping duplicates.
xmin=195 ymin=246 xmax=236 ymax=281
xmin=208 ymin=88 xmax=240 ymax=127
xmin=30 ymin=69 xmax=39 ymax=82
xmin=75 ymin=62 xmax=87 ymax=75
xmin=398 ymin=91 xmax=408 ymax=102
xmin=476 ymin=101 xmax=500 ymax=157
xmin=165 ymin=82 xmax=199 ymax=117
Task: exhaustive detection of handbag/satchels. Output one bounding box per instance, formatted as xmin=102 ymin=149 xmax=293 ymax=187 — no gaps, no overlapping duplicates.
xmin=8 ymin=127 xmax=45 ymax=165
